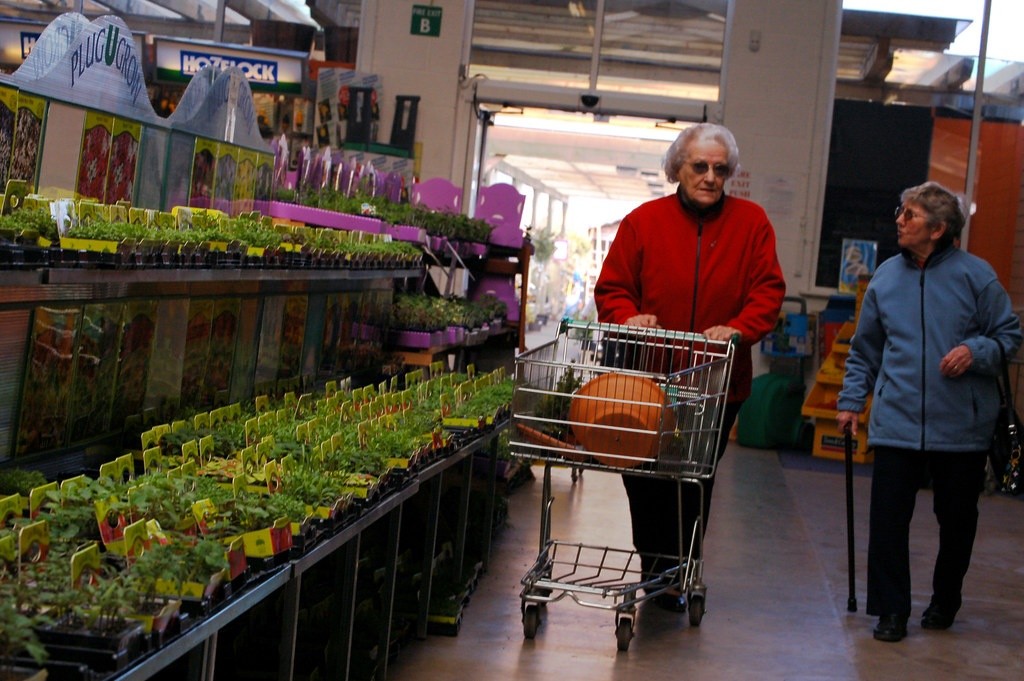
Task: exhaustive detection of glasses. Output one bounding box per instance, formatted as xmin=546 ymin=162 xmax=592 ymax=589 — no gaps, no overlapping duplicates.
xmin=895 ymin=206 xmax=929 ymax=221
xmin=682 ymin=161 xmax=729 ymax=177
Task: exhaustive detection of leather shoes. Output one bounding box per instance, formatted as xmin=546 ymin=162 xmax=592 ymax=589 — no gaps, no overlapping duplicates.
xmin=642 ymin=583 xmax=686 ymax=612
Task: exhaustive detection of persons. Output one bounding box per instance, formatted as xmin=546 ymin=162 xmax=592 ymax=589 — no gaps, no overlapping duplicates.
xmin=835 ymin=179 xmax=1024 ymax=644
xmin=595 ymin=120 xmax=787 ymax=614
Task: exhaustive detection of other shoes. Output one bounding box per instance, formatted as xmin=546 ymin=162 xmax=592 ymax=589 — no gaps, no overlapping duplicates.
xmin=873 ymin=614 xmax=907 ymax=641
xmin=921 ymin=593 xmax=962 ymax=629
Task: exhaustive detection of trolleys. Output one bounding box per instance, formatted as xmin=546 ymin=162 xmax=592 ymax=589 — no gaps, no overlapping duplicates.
xmin=503 ymin=317 xmax=740 ymax=652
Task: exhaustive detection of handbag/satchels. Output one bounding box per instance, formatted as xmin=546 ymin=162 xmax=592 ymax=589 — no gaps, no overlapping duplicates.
xmin=983 ymin=337 xmax=1024 ymax=493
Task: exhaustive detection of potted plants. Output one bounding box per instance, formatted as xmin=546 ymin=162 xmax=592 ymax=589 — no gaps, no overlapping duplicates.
xmin=0 ymin=179 xmax=584 ymax=681
